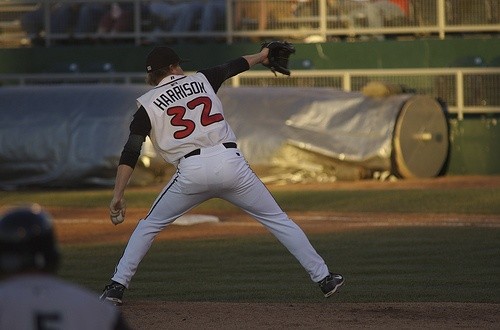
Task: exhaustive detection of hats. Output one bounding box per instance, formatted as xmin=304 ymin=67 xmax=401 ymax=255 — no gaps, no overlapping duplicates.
xmin=146 ymin=47 xmax=192 ymax=73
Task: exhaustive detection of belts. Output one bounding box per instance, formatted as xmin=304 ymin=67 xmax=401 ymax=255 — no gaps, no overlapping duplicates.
xmin=184 ymin=142 xmax=237 ymax=158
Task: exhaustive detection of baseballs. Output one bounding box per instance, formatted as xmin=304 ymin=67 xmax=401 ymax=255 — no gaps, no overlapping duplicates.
xmin=110 ymin=210 xmax=125 ymax=225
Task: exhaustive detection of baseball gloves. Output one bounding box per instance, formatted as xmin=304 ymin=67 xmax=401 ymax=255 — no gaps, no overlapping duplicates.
xmin=259 ymin=40 xmax=296 ymax=77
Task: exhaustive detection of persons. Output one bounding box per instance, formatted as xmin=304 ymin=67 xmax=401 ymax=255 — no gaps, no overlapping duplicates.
xmin=19 ymin=0 xmax=415 ymax=43
xmin=97 ymin=41 xmax=345 ymax=305
xmin=0 ymin=206 xmax=130 ymax=330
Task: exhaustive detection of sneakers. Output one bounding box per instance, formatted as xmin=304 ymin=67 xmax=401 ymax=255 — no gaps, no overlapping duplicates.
xmin=319 ymin=272 xmax=344 ymax=298
xmin=96 ymin=284 xmax=124 ymax=305
